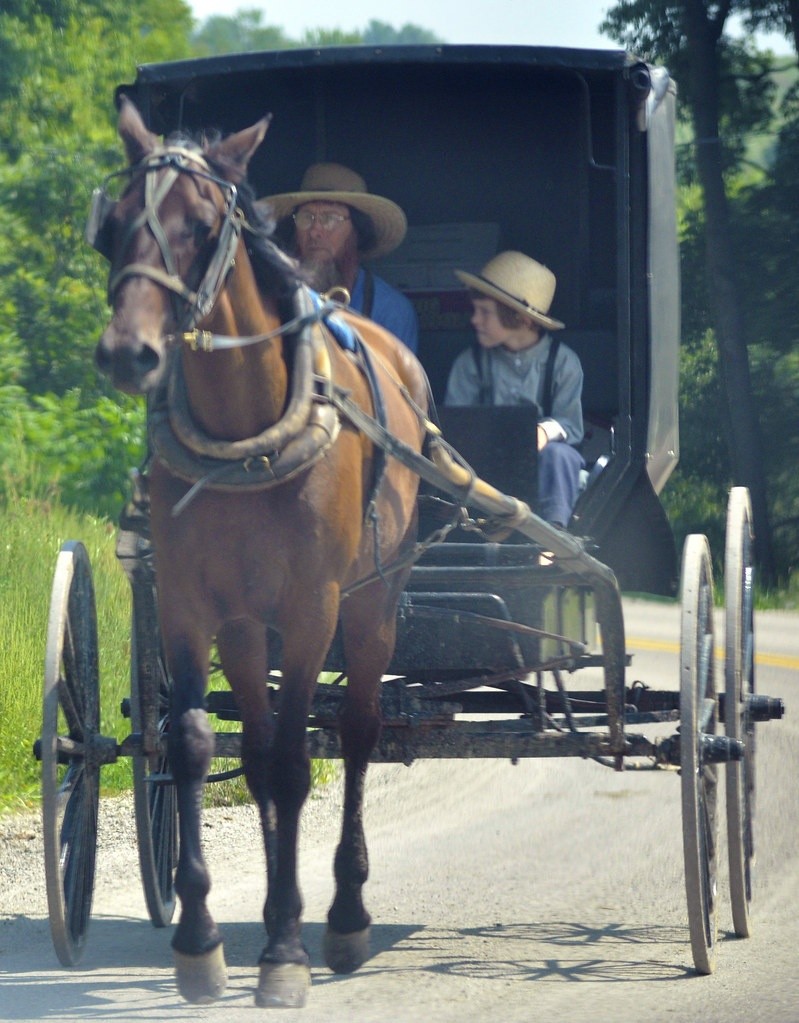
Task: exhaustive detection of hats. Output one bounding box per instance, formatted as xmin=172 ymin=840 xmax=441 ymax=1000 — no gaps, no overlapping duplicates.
xmin=458 ymin=245 xmax=569 ymax=336
xmin=243 ymin=165 xmax=409 ymax=260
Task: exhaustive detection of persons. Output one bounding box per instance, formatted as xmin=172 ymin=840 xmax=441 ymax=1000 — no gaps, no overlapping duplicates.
xmin=249 ymin=162 xmax=420 ymax=366
xmin=441 ymin=248 xmax=587 ymax=571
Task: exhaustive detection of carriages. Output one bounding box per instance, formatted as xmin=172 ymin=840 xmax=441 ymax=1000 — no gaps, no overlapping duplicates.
xmin=29 ymin=23 xmax=781 ymax=1008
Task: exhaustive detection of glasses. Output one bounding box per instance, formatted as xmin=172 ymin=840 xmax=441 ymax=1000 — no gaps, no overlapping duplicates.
xmin=289 ymin=208 xmax=354 ymax=234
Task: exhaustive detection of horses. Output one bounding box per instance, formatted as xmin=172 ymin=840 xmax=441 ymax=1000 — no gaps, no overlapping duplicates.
xmin=91 ymin=92 xmax=433 ymax=1010
